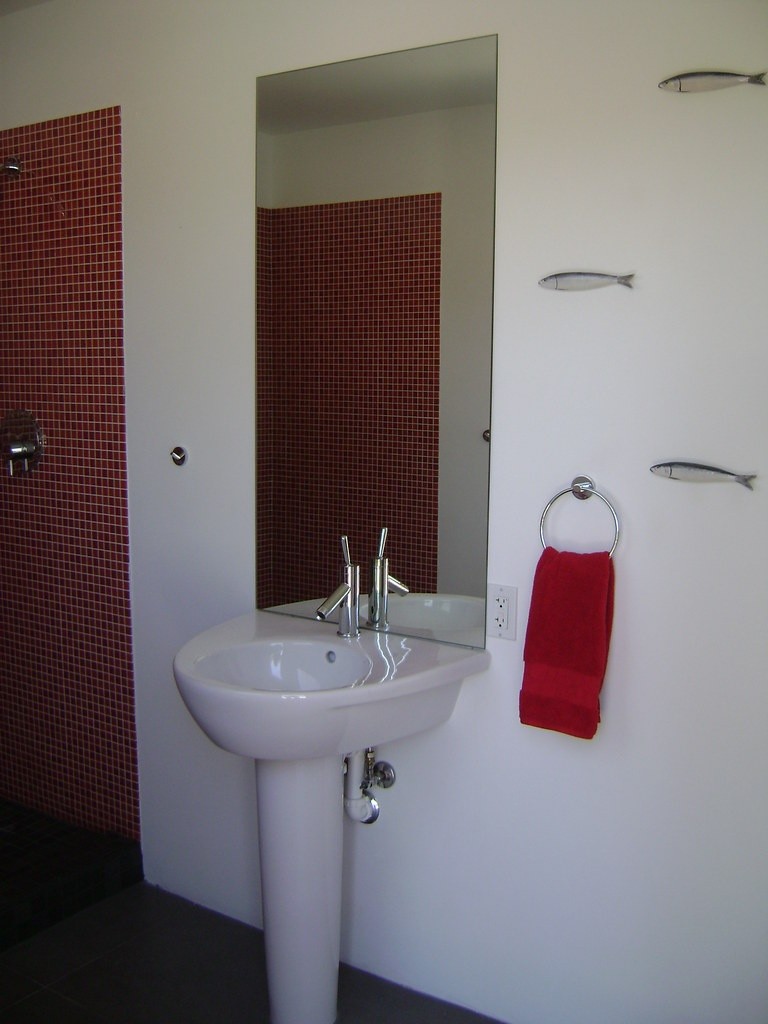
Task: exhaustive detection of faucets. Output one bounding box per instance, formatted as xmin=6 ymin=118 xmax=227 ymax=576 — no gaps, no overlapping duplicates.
xmin=365 ymin=527 xmax=409 ymax=628
xmin=314 ymin=535 xmax=361 ymax=638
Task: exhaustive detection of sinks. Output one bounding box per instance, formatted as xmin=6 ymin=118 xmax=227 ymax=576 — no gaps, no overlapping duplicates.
xmin=172 ymin=609 xmax=491 ymax=761
xmin=265 ymin=592 xmax=486 ymax=650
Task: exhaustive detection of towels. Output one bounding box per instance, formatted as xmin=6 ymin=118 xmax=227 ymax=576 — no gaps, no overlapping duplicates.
xmin=518 ymin=546 xmax=616 ymax=743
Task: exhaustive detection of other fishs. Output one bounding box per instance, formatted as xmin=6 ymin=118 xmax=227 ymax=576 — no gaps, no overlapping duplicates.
xmin=657 ymin=70 xmax=768 ymax=94
xmin=538 ymin=271 xmax=636 ymax=291
xmin=649 ymin=461 xmax=757 ymax=492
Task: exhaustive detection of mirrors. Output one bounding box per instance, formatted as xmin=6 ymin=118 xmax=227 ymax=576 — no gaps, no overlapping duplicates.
xmin=253 ymin=31 xmax=499 ymax=653
